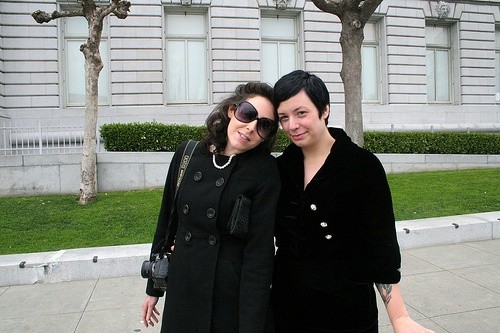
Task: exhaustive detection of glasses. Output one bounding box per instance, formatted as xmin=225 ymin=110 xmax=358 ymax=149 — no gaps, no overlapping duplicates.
xmin=233 ymin=101 xmax=280 ymax=141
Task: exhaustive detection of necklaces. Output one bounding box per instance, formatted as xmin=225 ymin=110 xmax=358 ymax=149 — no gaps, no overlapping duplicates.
xmin=212 ymin=152 xmax=235 ymax=170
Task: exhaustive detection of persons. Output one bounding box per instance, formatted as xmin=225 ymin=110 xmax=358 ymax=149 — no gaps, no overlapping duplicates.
xmin=274 ymin=71 xmax=435 ymax=333
xmin=138 ymin=80 xmax=282 ymax=333
